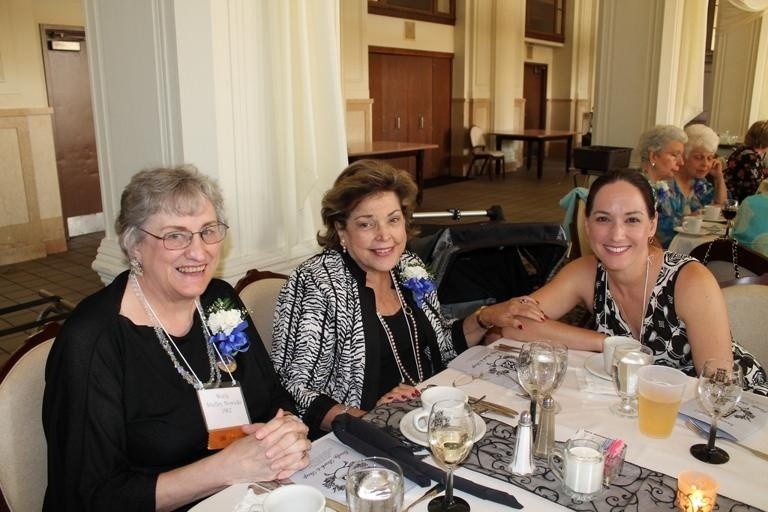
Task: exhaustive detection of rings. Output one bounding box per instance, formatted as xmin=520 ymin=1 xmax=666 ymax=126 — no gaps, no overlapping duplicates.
xmin=520 ymin=298 xmax=528 ymax=305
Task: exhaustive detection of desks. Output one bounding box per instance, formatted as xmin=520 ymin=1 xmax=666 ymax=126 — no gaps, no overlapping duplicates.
xmin=347 ymin=140 xmax=438 ymax=207
xmin=496 ymin=129 xmax=576 ymax=179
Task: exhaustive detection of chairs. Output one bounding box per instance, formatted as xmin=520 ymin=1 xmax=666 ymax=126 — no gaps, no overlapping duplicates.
xmin=722 ymin=284 xmax=768 ymax=373
xmin=466 ymin=125 xmax=506 ymax=181
xmin=690 ymin=239 xmax=767 ymax=285
xmin=234 ymin=269 xmax=290 ymax=366
xmin=1 ymin=320 xmax=57 ymax=511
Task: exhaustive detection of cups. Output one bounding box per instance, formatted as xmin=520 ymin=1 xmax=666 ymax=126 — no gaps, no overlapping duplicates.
xmin=548 ymin=435 xmax=608 ymax=502
xmin=247 ymin=483 xmax=327 ymax=511
xmin=512 ymin=340 xmax=556 ymax=444
xmin=682 ymin=214 xmax=704 ymax=234
xmin=346 ymin=456 xmax=405 ymax=510
xmin=699 ymin=205 xmax=721 ymax=220
xmin=413 ymin=386 xmax=469 ymax=433
xmin=603 ymin=335 xmax=653 ymax=375
xmin=690 ymin=359 xmax=744 ymax=463
xmin=636 ymin=364 xmax=689 ymax=441
xmin=428 ymin=400 xmax=476 ymax=512
xmin=535 ymin=340 xmax=568 ymax=416
xmin=718 ymin=199 xmax=738 ymax=238
xmin=675 ymin=469 xmax=720 ymax=511
xmin=572 ymin=427 xmax=628 ymax=486
xmin=609 ymin=344 xmax=653 ymax=418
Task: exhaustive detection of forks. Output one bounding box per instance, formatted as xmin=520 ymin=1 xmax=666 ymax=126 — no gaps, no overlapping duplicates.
xmin=684 ymin=416 xmax=768 ymax=461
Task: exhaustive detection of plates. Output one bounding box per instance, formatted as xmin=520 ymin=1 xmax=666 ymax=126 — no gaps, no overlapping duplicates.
xmin=399 ymin=406 xmax=487 ymax=448
xmin=696 ymin=215 xmax=728 ymax=223
xmin=584 ymin=352 xmax=656 ymax=381
xmin=673 ymin=226 xmax=711 ymax=236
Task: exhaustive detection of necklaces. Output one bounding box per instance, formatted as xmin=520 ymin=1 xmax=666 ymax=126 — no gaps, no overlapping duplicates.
xmin=367 ymin=266 xmax=425 ymax=392
xmin=600 ymin=259 xmax=654 ymax=353
xmin=128 ymin=269 xmax=218 ymax=394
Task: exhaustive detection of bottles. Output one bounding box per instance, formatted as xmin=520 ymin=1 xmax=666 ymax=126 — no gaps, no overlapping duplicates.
xmin=533 ymin=396 xmax=555 ymax=459
xmin=506 ymin=411 xmax=537 ymax=474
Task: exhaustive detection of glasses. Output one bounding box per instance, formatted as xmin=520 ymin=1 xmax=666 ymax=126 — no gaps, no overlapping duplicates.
xmin=133 ymin=220 xmax=230 ymax=251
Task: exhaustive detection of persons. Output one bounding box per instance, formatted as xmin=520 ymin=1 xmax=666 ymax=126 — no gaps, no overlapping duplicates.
xmin=503 ymin=168 xmax=766 ymax=401
xmin=634 ymin=125 xmax=691 ymax=249
xmin=39 ymin=162 xmax=310 ymax=511
xmin=269 ymin=160 xmax=546 ymax=428
xmin=729 ymin=182 xmax=768 ymax=262
xmin=718 ymin=119 xmax=768 ymax=203
xmin=679 ymin=123 xmax=732 ymax=215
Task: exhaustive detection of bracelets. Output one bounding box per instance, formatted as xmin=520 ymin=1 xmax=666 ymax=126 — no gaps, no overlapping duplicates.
xmin=472 ymin=305 xmax=494 ymax=330
